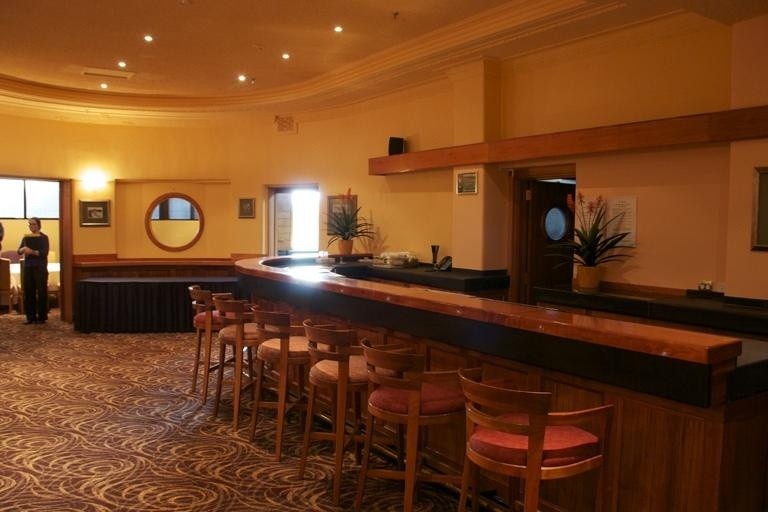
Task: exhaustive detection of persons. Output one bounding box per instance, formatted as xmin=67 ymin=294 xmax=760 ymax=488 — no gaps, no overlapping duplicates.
xmin=17 ymin=217 xmax=49 ymax=324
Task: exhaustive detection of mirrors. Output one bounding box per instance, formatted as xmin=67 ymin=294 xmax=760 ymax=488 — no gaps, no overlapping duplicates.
xmin=144 ymin=191 xmax=205 ymax=253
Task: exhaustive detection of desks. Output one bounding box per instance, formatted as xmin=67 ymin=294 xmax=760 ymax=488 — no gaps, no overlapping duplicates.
xmin=234 ymin=252 xmax=768 ymax=512
xmin=74 ymin=275 xmax=243 ymax=335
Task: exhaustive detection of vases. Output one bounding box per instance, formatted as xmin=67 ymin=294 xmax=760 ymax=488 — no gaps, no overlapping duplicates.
xmin=577 ymin=262 xmax=604 ymax=292
xmin=337 ymin=239 xmax=351 ymax=257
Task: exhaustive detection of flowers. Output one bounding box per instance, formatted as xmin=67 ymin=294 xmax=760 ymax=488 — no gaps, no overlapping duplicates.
xmin=323 ymin=190 xmax=382 ymax=246
xmin=549 ymin=192 xmax=635 ymax=264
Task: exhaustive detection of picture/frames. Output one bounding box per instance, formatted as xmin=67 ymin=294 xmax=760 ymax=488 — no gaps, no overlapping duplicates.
xmin=456 ymin=171 xmax=478 ymax=195
xmin=237 ymin=197 xmax=255 ymax=220
xmin=79 ymin=199 xmax=111 ymax=229
xmin=749 ymin=164 xmax=768 ymax=251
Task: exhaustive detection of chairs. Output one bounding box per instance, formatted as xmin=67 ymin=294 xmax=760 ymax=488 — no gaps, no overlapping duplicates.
xmin=0 ymin=250 xmax=61 ymax=314
xmin=352 ymin=337 xmax=483 ymax=512
xmin=455 ymin=365 xmax=614 ymax=512
xmin=214 ymin=294 xmax=292 ymax=428
xmin=298 ymin=319 xmax=407 ymax=506
xmin=187 ymin=282 xmax=256 ymax=407
xmin=248 ymin=305 xmax=346 ymax=466
